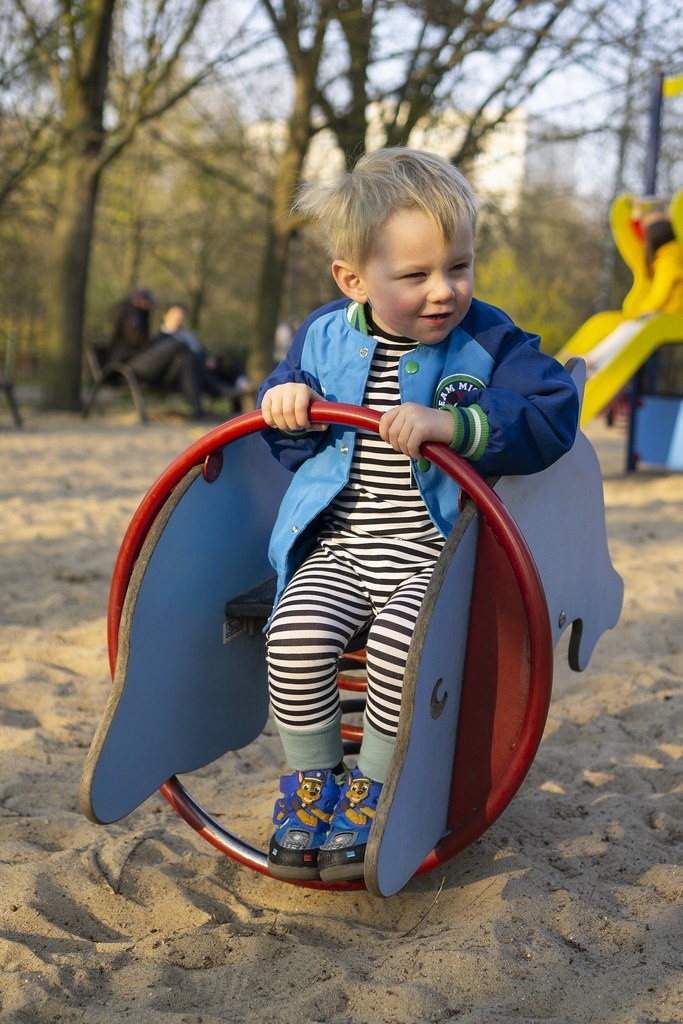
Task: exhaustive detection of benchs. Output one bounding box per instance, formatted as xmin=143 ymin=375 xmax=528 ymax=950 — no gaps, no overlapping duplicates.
xmin=82 ymin=340 xmax=242 ymax=423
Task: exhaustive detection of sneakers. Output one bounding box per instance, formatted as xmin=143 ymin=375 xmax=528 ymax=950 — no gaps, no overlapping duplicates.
xmin=266 ymin=761 xmax=350 ymax=879
xmin=316 ymin=765 xmax=384 ymax=882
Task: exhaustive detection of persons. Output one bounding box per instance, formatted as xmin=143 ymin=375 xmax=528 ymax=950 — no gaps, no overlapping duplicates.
xmin=257 ymin=148 xmax=582 ymax=884
xmin=108 ymin=289 xmax=302 ymax=420
xmin=582 ymin=219 xmax=683 ymax=379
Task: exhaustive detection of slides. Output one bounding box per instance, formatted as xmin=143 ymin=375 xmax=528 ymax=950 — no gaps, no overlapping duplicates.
xmin=555 ymin=302 xmax=682 ymax=429
